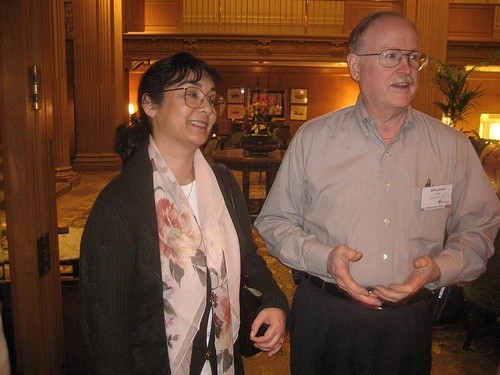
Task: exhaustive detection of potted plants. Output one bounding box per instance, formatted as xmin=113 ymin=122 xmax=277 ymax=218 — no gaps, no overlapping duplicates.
xmin=241 ymin=101 xmax=282 ymax=135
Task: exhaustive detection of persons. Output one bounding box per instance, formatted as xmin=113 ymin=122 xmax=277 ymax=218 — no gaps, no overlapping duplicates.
xmin=79 ymin=52 xmax=293 ymax=375
xmin=254 ymin=11 xmax=500 ymax=375
xmin=113 ymin=113 xmax=143 ymax=168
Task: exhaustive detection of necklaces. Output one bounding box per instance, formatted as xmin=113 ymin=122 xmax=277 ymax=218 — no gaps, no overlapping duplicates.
xmin=187 ymin=169 xmax=194 ymax=199
xmin=382 ymin=137 xmax=393 ymax=140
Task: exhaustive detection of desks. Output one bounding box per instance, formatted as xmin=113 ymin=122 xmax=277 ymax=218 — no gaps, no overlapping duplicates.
xmin=210 ymin=149 xmax=287 ymax=216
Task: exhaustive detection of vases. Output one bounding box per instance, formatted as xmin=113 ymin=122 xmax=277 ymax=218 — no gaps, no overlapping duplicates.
xmin=240 ymin=133 xmax=282 ymax=155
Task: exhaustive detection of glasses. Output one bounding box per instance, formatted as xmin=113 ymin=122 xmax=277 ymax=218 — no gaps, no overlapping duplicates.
xmin=147 ymin=86 xmax=227 ymax=117
xmin=355 ymin=48 xmax=430 ymax=71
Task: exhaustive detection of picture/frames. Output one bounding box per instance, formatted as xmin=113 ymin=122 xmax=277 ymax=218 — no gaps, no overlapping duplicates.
xmin=249 ymin=89 xmax=284 ymax=118
xmin=226 ymin=86 xmax=245 ymax=120
xmin=289 ymin=88 xmax=308 ymax=105
xmin=289 ymin=105 xmax=308 ymax=121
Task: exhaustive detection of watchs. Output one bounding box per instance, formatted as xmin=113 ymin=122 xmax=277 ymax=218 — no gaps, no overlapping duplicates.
xmin=424 ymin=280 xmax=439 ymax=290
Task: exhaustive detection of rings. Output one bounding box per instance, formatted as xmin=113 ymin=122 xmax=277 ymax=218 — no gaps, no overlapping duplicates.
xmin=278 ymin=341 xmax=282 ymax=345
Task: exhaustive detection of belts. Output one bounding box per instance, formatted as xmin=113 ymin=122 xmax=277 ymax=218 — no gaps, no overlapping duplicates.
xmin=299 ymin=271 xmax=435 ymax=310
